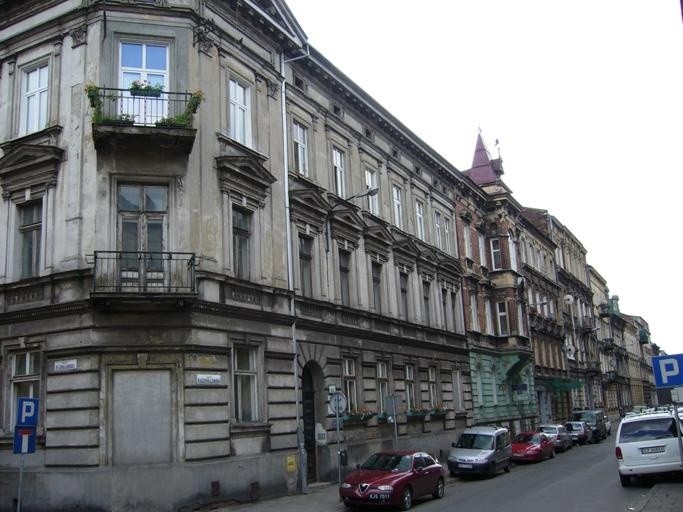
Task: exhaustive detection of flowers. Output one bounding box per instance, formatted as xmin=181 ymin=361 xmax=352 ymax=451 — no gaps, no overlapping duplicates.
xmin=131 ymin=79 xmax=166 ymax=90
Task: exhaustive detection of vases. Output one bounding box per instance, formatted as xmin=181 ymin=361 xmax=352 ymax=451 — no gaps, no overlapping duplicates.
xmin=131 ymin=88 xmax=161 ymax=96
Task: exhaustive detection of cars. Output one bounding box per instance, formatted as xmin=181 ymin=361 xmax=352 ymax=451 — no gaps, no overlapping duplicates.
xmin=339 ymin=451 xmax=445 ymax=511
xmin=445 ymin=408 xmax=614 ymax=481
xmin=614 ymin=403 xmax=683 ymax=490
